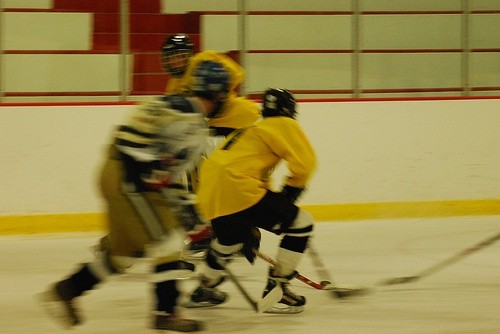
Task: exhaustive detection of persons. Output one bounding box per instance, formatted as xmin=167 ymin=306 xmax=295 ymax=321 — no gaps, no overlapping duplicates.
xmin=50 ymin=51 xmax=244 ymax=333
xmin=160 ymin=33 xmax=261 ymax=252
xmin=179 ymin=87 xmax=318 ymax=307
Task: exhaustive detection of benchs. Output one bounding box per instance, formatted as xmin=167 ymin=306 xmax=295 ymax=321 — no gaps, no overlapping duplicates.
xmin=0 ymin=0 xmax=133 ymax=96
xmin=159 ymin=0 xmax=500 ymax=91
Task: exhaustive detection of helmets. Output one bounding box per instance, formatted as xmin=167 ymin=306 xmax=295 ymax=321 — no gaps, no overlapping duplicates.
xmin=160 ymin=34 xmax=194 ymax=78
xmin=182 ymin=51 xmax=244 ymax=118
xmin=262 ymin=87 xmax=297 ymax=119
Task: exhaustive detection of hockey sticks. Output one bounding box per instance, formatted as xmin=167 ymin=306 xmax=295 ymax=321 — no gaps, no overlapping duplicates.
xmin=256 ymin=250 xmax=332 ymax=290
xmin=305 ymin=238 xmax=377 ymax=301
xmin=377 ymin=232 xmax=500 ymax=287
xmin=209 ymin=247 xmax=283 ymax=315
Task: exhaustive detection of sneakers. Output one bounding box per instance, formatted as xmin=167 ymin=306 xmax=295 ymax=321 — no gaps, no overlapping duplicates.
xmin=42 ymin=284 xmax=81 ymax=326
xmin=151 ymin=314 xmax=201 ymax=332
xmin=255 ymin=269 xmax=306 ymax=313
xmin=185 ymin=286 xmax=228 ymax=308
